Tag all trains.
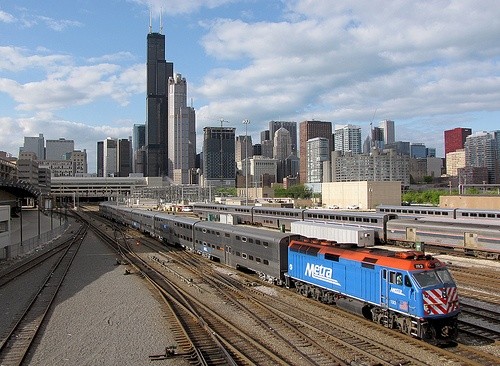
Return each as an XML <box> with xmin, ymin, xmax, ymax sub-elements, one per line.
<box><xmin>98</xmin><ymin>201</ymin><xmax>460</xmax><ymax>345</ymax></box>
<box><xmin>290</xmin><ymin>219</ymin><xmax>374</xmax><ymax>252</ymax></box>
<box><xmin>376</xmin><ymin>204</ymin><xmax>499</xmax><ymax>225</ymax></box>
<box><xmin>192</xmin><ymin>203</ymin><xmax>500</xmax><ymax>276</ymax></box>
